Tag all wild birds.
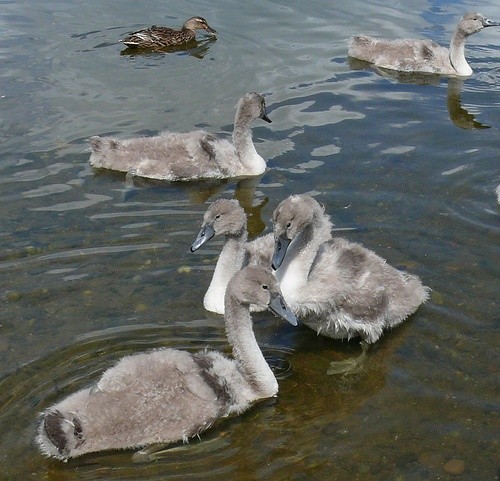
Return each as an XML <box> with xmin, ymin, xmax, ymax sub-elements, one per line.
<box><xmin>268</xmin><ymin>193</ymin><xmax>433</xmax><ymax>374</ymax></box>
<box><xmin>119</xmin><ymin>16</ymin><xmax>217</xmax><ymax>51</ymax></box>
<box><xmin>190</xmin><ymin>198</ymin><xmax>278</xmax><ymax>314</ymax></box>
<box><xmin>349</xmin><ymin>58</ymin><xmax>493</xmax><ymax>130</ymax></box>
<box><xmin>33</xmin><ymin>266</ymin><xmax>298</xmax><ymax>463</ymax></box>
<box><xmin>347</xmin><ymin>11</ymin><xmax>499</xmax><ymax>77</ymax></box>
<box><xmin>87</xmin><ymin>92</ymin><xmax>273</xmax><ymax>182</ymax></box>
